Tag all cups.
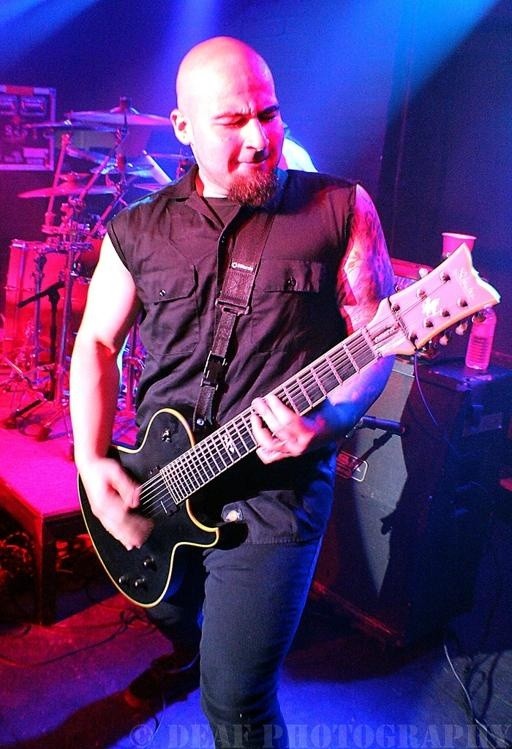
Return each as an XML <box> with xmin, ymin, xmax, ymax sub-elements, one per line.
<box><xmin>441</xmin><ymin>233</ymin><xmax>476</xmax><ymax>258</ymax></box>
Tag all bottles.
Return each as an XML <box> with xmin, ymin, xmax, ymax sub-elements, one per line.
<box><xmin>466</xmin><ymin>304</ymin><xmax>498</xmax><ymax>371</ymax></box>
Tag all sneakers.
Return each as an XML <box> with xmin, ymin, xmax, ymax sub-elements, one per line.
<box><xmin>123</xmin><ymin>649</ymin><xmax>200</xmax><ymax>709</ymax></box>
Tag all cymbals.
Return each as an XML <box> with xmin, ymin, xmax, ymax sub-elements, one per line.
<box><xmin>147</xmin><ymin>152</ymin><xmax>194</xmax><ymax>161</ymax></box>
<box><xmin>17</xmin><ymin>182</ymin><xmax>119</xmax><ymax>199</ymax></box>
<box><xmin>90</xmin><ymin>163</ymin><xmax>155</xmax><ymax>175</ymax></box>
<box><xmin>26</xmin><ymin>107</ymin><xmax>172</xmax><ymax>129</ymax></box>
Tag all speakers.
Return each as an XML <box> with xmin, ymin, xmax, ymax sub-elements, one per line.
<box><xmin>316</xmin><ymin>358</ymin><xmax>512</xmax><ymax>645</ymax></box>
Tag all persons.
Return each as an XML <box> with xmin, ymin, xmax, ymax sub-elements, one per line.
<box><xmin>69</xmin><ymin>35</ymin><xmax>395</xmax><ymax>749</ymax></box>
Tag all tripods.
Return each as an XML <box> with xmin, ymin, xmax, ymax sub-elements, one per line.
<box><xmin>0</xmin><ymin>156</ymin><xmax>116</xmax><ymax>462</ymax></box>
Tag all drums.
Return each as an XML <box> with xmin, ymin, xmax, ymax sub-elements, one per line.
<box><xmin>4</xmin><ymin>238</ymin><xmax>79</xmax><ymax>312</ymax></box>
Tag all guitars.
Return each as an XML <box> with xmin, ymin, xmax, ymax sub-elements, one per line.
<box><xmin>77</xmin><ymin>244</ymin><xmax>501</xmax><ymax>609</ymax></box>
